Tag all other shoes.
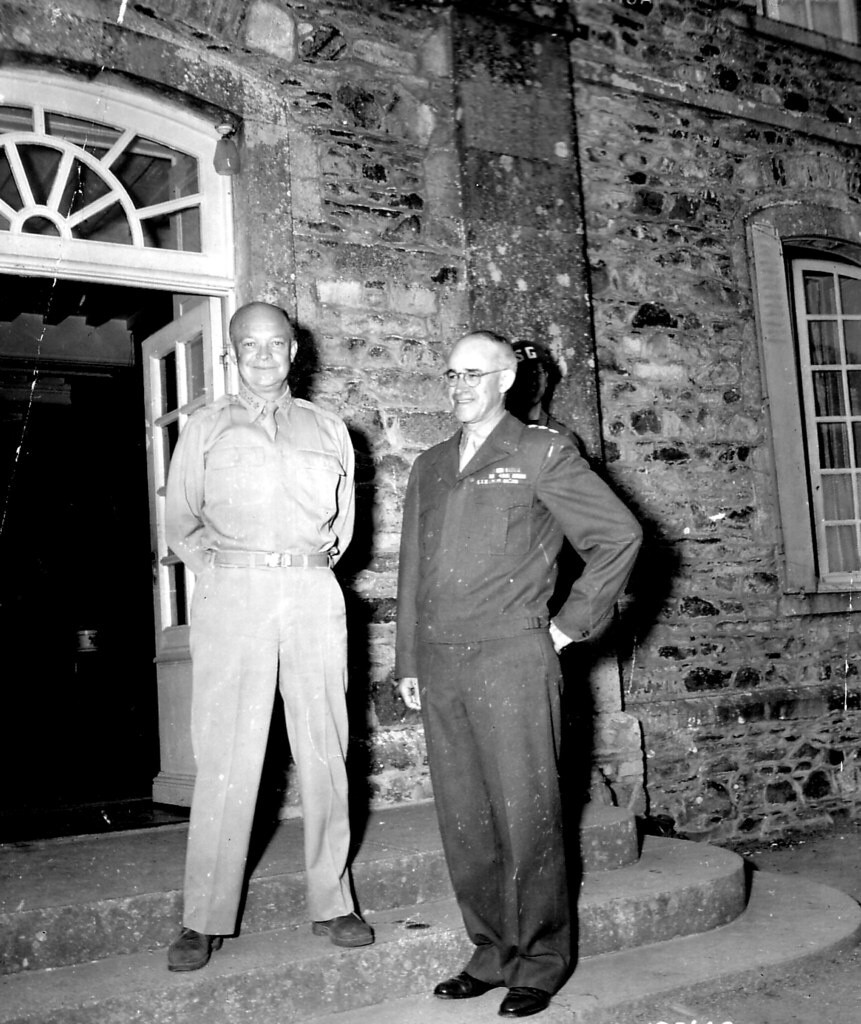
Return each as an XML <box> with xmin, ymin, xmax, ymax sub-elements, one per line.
<box><xmin>312</xmin><ymin>914</ymin><xmax>374</xmax><ymax>946</ymax></box>
<box><xmin>166</xmin><ymin>928</ymin><xmax>219</xmax><ymax>972</ymax></box>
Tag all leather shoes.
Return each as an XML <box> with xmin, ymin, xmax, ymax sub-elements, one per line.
<box><xmin>498</xmin><ymin>987</ymin><xmax>548</xmax><ymax>1017</ymax></box>
<box><xmin>432</xmin><ymin>971</ymin><xmax>493</xmax><ymax>999</ymax></box>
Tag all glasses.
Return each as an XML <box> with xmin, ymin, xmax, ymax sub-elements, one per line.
<box><xmin>441</xmin><ymin>369</ymin><xmax>505</xmax><ymax>387</ymax></box>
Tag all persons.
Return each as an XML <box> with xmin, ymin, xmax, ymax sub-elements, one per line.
<box><xmin>395</xmin><ymin>332</ymin><xmax>645</xmax><ymax>1014</ymax></box>
<box><xmin>165</xmin><ymin>302</ymin><xmax>374</xmax><ymax>973</ymax></box>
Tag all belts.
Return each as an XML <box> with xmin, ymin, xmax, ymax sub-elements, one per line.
<box><xmin>213</xmin><ymin>551</ymin><xmax>327</xmax><ymax>567</ymax></box>
